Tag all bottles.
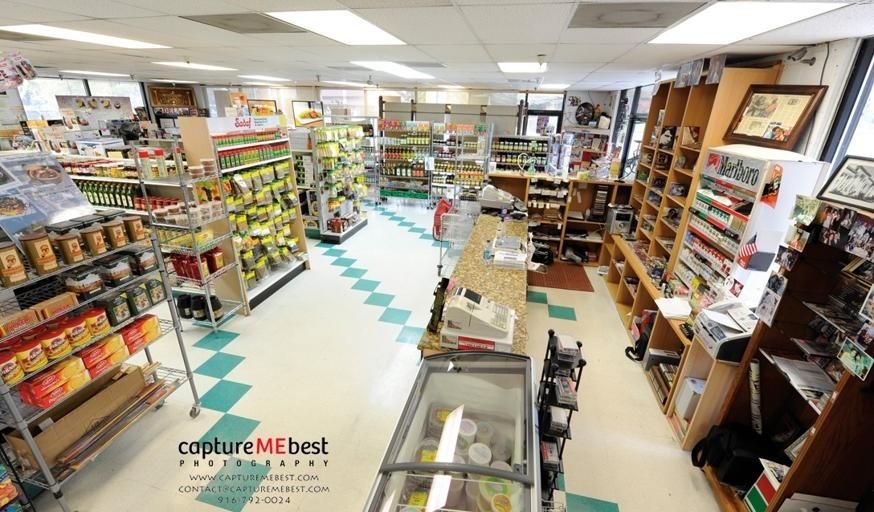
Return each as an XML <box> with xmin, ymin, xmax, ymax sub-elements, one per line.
<box><xmin>484</xmin><ymin>239</ymin><xmax>492</xmax><ymax>265</ymax></box>
<box><xmin>73</xmin><ymin>181</ymin><xmax>142</xmax><ymax>211</ymax></box>
<box><xmin>211</xmin><ymin>128</ymin><xmax>291</xmax><ymax>170</ymax></box>
<box><xmin>138</xmin><ymin>148</ymin><xmax>185</xmax><ymax>180</ymax></box>
<box><xmin>383</xmin><ymin>129</ymin><xmax>547</xmax><ymax>186</ymax></box>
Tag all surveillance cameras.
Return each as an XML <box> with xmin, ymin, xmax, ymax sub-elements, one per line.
<box><xmin>787</xmin><ymin>47</ymin><xmax>807</xmax><ymax>61</ymax></box>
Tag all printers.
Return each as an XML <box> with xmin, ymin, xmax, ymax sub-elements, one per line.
<box><xmin>691</xmin><ymin>298</ymin><xmax>760</xmax><ymax>362</ymax></box>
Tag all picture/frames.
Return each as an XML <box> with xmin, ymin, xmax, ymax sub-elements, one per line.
<box><xmin>148</xmin><ymin>86</ymin><xmax>199</xmax><ymax>108</ymax></box>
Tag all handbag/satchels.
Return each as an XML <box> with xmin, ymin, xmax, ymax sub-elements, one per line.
<box><xmin>636</xmin><ymin>322</ymin><xmax>654</xmax><ymax>359</ymax></box>
<box><xmin>565</xmin><ymin>244</ymin><xmax>589</xmax><ymax>264</ymax></box>
<box><xmin>532</xmin><ymin>247</ymin><xmax>553</xmax><ymax>265</ymax></box>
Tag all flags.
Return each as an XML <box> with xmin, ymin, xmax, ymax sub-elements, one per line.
<box><xmin>737</xmin><ymin>234</ymin><xmax>758</xmax><ymax>258</ymax></box>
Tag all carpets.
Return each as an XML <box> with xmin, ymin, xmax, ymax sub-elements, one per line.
<box><xmin>526</xmin><ymin>260</ymin><xmax>594</xmax><ymax>293</ymax></box>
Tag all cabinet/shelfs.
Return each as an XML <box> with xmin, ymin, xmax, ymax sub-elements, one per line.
<box><xmin>537</xmin><ymin>328</ymin><xmax>586</xmax><ymax>507</ymax></box>
<box><xmin>373</xmin><ymin>120</ymin><xmax>633</xmax><ymax>264</ymax></box>
<box><xmin>598</xmin><ymin>63</ymin><xmax>874</xmax><ymax>511</ymax></box>
<box><xmin>0</xmin><ymin>114</ymin><xmax>366</xmax><ymax>511</ymax></box>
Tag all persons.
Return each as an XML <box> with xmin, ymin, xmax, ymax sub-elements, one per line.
<box><xmin>764</xmin><ymin>126</ymin><xmax>789</xmax><ymax>142</ymax></box>
<box><xmin>659</xmin><ymin>126</ymin><xmax>676</xmax><ymax>150</ymax></box>
<box><xmin>753</xmin><ymin>210</ymin><xmax>874</xmax><ymax>378</ymax></box>
<box><xmin>665</xmin><ymin>209</ymin><xmax>677</xmax><ymax>219</ymax></box>
<box><xmin>746</xmin><ymin>96</ymin><xmax>778</xmax><ymax>118</ymax></box>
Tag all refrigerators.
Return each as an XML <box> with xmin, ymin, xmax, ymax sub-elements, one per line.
<box><xmin>366</xmin><ymin>349</ymin><xmax>538</xmax><ymax>512</ymax></box>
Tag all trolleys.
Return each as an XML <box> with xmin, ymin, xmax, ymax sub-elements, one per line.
<box><xmin>437</xmin><ymin>176</ymin><xmax>486</xmax><ymax>276</ymax></box>
<box><xmin>374</xmin><ymin>162</ymin><xmax>430</xmax><ymax>209</ymax></box>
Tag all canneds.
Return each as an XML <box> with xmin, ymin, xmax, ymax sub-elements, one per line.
<box><xmin>0</xmin><ymin>241</ymin><xmax>29</xmax><ymax>289</ymax></box>
<box><xmin>101</xmin><ymin>220</ymin><xmax>127</xmax><ymax>249</ymax></box>
<box><xmin>122</xmin><ymin>216</ymin><xmax>145</xmax><ymax>242</ymax></box>
<box><xmin>17</xmin><ymin>233</ymin><xmax>59</xmax><ymax>275</ymax></box>
<box><xmin>54</xmin><ymin>234</ymin><xmax>85</xmax><ymax>265</ymax></box>
<box><xmin>491</xmin><ymin>141</ymin><xmax>547</xmax><ymax>164</ymax></box>
<box><xmin>1</xmin><ymin>307</ymin><xmax>111</xmax><ymax>383</ymax></box>
<box><xmin>133</xmin><ymin>196</ymin><xmax>180</xmax><ymax>212</ymax></box>
<box><xmin>79</xmin><ymin>226</ymin><xmax>108</xmax><ymax>257</ymax></box>
